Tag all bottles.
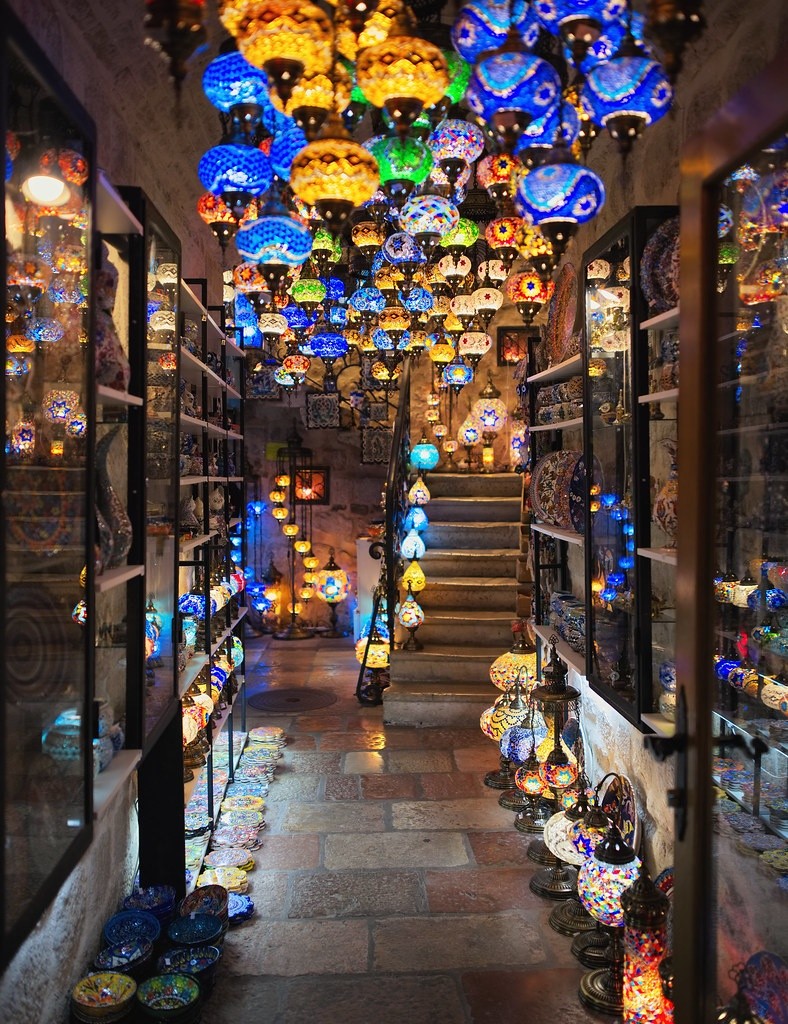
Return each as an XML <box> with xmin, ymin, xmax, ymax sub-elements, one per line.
<box><xmin>731</xmin><ymin>569</ymin><xmax>758</xmax><ymax>607</ymax></box>
<box><xmin>728</xmin><ymin>658</ymin><xmax>757</xmax><ymax>687</ymax></box>
<box><xmin>761</xmin><ymin>670</ymin><xmax>788</xmax><ymax>710</ymax></box>
<box><xmin>712</xmin><ymin>567</ymin><xmax>724</xmax><ymax>585</ymax></box>
<box><xmin>743</xmin><ymin>656</ymin><xmax>777</xmax><ymax>697</ymax></box>
<box><xmin>763</xmin><ymin>627</ymin><xmax>781</xmax><ymax>643</ymax></box>
<box><xmin>715</xmin><ymin>569</ymin><xmax>739</xmax><ymax>603</ymax></box>
<box><xmin>751</xmin><ymin>618</ymin><xmax>771</xmax><ymax>643</ymax></box>
<box><xmin>715</xmin><ymin>649</ymin><xmax>741</xmax><ymax>681</ymax></box>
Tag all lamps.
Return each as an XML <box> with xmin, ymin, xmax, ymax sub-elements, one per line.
<box><xmin>0</xmin><ymin>0</ymin><xmax>788</xmax><ymax>1024</ymax></box>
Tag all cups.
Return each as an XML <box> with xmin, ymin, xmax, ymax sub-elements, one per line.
<box><xmin>43</xmin><ymin>700</ymin><xmax>125</xmax><ymax>780</ymax></box>
<box><xmin>537</xmin><ymin>375</ymin><xmax>586</xmax><ymax>423</ymax></box>
<box><xmin>659</xmin><ymin>331</ymin><xmax>681</xmax><ymax>389</ymax></box>
<box><xmin>658</xmin><ymin>661</ymin><xmax>677</xmax><ymax>722</ymax></box>
<box><xmin>549</xmin><ymin>590</ymin><xmax>616</xmax><ymax>676</ymax></box>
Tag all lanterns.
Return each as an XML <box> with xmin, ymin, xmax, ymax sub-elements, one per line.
<box><xmin>143</xmin><ymin>0</ymin><xmax>788</xmax><ymax>1024</ymax></box>
<box><xmin>5</xmin><ymin>129</ymin><xmax>92</xmax><ymax>455</ymax></box>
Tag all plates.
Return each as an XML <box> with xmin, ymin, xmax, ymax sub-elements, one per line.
<box><xmin>542</xmin><ymin>262</ymin><xmax>582</xmax><ymax>366</ymax></box>
<box><xmin>640</xmin><ymin>215</ymin><xmax>680</xmax><ymax>311</ymax></box>
<box><xmin>530</xmin><ymin>451</ymin><xmax>606</xmax><ymax>535</ymax></box>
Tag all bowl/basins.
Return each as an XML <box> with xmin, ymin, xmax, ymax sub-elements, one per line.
<box><xmin>71</xmin><ymin>884</ymin><xmax>229</xmax><ymax>1024</ymax></box>
